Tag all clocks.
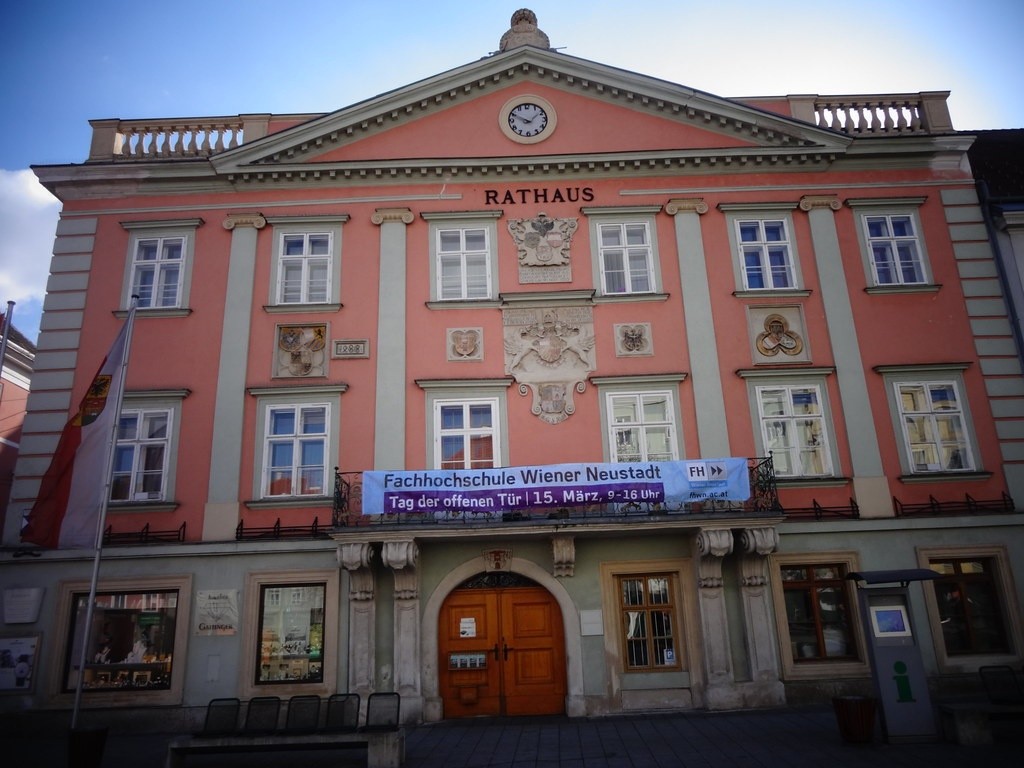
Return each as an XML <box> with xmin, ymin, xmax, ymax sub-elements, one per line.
<box><xmin>498</xmin><ymin>94</ymin><xmax>558</xmax><ymax>144</ymax></box>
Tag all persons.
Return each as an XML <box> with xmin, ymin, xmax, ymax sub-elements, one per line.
<box><xmin>0</xmin><ymin>649</ymin><xmax>16</xmax><ymax>669</ymax></box>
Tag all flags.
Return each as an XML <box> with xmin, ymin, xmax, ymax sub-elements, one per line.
<box><xmin>20</xmin><ymin>308</ymin><xmax>134</xmax><ymax>548</ymax></box>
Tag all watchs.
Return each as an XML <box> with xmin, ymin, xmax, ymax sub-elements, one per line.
<box><xmin>15</xmin><ymin>654</ymin><xmax>29</xmax><ymax>687</ymax></box>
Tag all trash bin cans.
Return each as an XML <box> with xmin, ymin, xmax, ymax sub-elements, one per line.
<box><xmin>833</xmin><ymin>695</ymin><xmax>878</xmax><ymax>745</ymax></box>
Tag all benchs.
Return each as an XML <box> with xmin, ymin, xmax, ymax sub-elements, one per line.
<box><xmin>936</xmin><ymin>664</ymin><xmax>1024</xmax><ymax>747</ymax></box>
<box><xmin>167</xmin><ymin>692</ymin><xmax>406</xmax><ymax>768</ymax></box>
